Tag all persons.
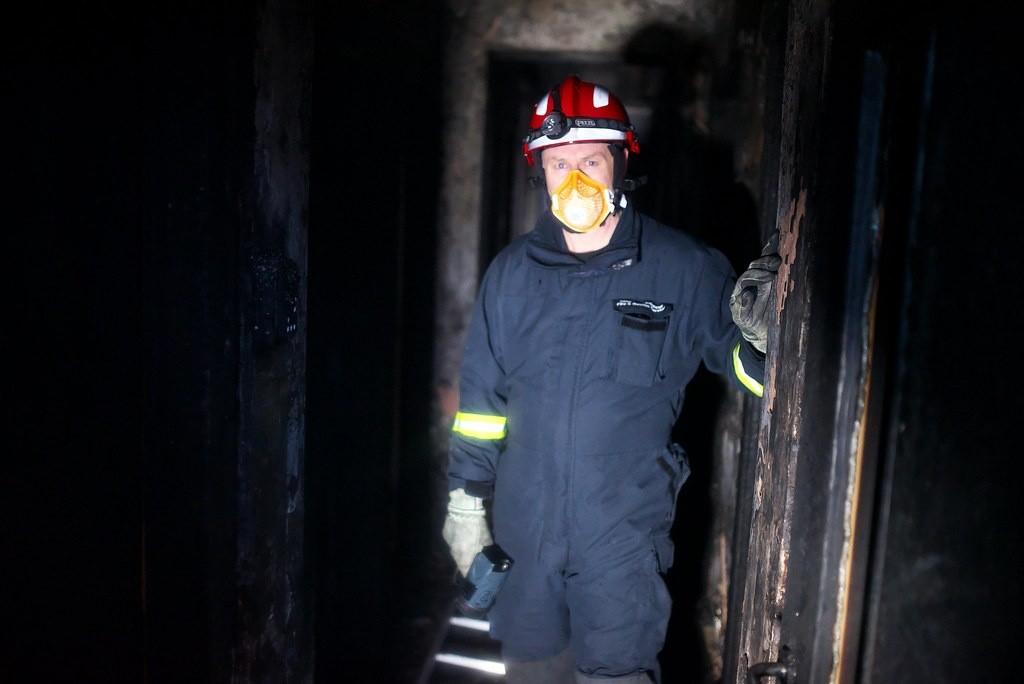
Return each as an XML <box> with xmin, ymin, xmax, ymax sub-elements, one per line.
<box><xmin>441</xmin><ymin>74</ymin><xmax>782</xmax><ymax>684</ymax></box>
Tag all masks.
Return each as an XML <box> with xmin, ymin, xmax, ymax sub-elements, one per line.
<box><xmin>551</xmin><ymin>169</ymin><xmax>616</xmax><ymax>234</ymax></box>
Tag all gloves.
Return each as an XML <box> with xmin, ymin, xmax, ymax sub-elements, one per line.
<box><xmin>730</xmin><ymin>230</ymin><xmax>783</xmax><ymax>351</ymax></box>
<box><xmin>441</xmin><ymin>486</ymin><xmax>494</xmax><ymax>583</ymax></box>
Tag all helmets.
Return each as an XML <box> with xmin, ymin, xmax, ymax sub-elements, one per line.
<box><xmin>523</xmin><ymin>75</ymin><xmax>639</xmax><ymax>165</ymax></box>
